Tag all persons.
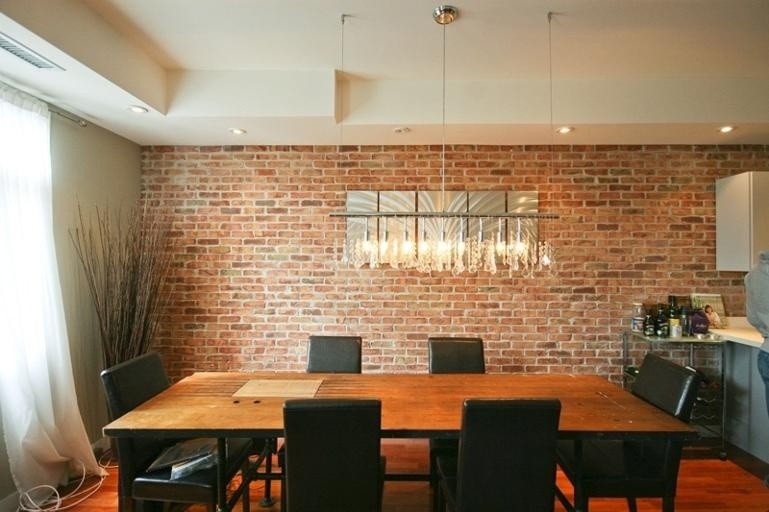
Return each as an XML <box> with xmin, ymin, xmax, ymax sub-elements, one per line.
<box><xmin>705</xmin><ymin>304</ymin><xmax>721</xmax><ymax>328</ymax></box>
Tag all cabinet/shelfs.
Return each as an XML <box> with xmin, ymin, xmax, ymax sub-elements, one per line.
<box><xmin>625</xmin><ymin>332</ymin><xmax>728</xmax><ymax>458</ymax></box>
<box><xmin>725</xmin><ymin>346</ymin><xmax>768</xmax><ymax>479</ymax></box>
<box><xmin>714</xmin><ymin>170</ymin><xmax>769</xmax><ymax>274</ymax></box>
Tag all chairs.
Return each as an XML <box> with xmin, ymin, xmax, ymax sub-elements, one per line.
<box><xmin>435</xmin><ymin>400</ymin><xmax>562</xmax><ymax>511</ymax></box>
<box><xmin>282</xmin><ymin>398</ymin><xmax>385</xmax><ymax>510</ymax></box>
<box><xmin>103</xmin><ymin>351</ymin><xmax>253</xmax><ymax>512</ymax></box>
<box><xmin>428</xmin><ymin>338</ymin><xmax>485</xmax><ymax>487</ymax></box>
<box><xmin>556</xmin><ymin>353</ymin><xmax>699</xmax><ymax>511</ymax></box>
<box><xmin>306</xmin><ymin>336</ymin><xmax>362</xmax><ymax>373</ymax></box>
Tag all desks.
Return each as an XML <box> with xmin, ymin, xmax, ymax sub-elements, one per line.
<box><xmin>104</xmin><ymin>372</ymin><xmax>698</xmax><ymax>511</ymax></box>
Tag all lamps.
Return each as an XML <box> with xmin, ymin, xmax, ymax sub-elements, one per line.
<box><xmin>331</xmin><ymin>5</ymin><xmax>557</xmax><ymax>279</ymax></box>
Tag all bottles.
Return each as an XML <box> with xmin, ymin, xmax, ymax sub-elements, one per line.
<box><xmin>631</xmin><ymin>295</ymin><xmax>692</xmax><ymax>338</ymax></box>
